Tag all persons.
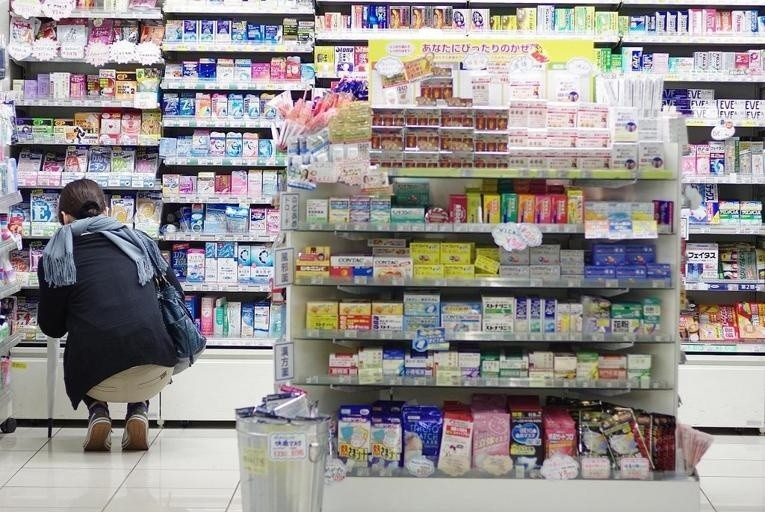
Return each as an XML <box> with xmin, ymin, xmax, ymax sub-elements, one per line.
<box><xmin>37</xmin><ymin>178</ymin><xmax>186</xmax><ymax>452</ymax></box>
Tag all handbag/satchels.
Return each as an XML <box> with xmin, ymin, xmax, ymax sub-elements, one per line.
<box><xmin>133</xmin><ymin>230</ymin><xmax>206</xmax><ymax>374</ymax></box>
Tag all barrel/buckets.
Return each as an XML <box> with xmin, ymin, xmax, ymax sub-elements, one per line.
<box><xmin>236</xmin><ymin>416</ymin><xmax>332</xmax><ymax>512</ymax></box>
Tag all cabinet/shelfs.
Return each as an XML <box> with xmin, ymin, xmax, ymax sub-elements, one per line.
<box><xmin>0</xmin><ymin>2</ymin><xmax>764</xmax><ymax>476</ymax></box>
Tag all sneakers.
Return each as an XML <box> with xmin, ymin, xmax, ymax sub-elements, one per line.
<box><xmin>122</xmin><ymin>410</ymin><xmax>149</xmax><ymax>450</ymax></box>
<box><xmin>83</xmin><ymin>407</ymin><xmax>112</xmax><ymax>451</ymax></box>
